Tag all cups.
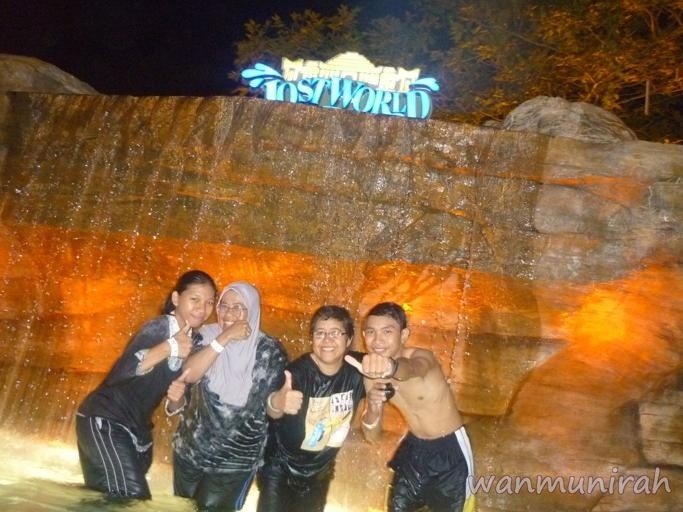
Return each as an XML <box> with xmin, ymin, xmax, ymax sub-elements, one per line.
<box><xmin>216</xmin><ymin>305</ymin><xmax>247</xmax><ymax>314</ymax></box>
<box><xmin>313</xmin><ymin>330</ymin><xmax>349</xmax><ymax>339</ymax></box>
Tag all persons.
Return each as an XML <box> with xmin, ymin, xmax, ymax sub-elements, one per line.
<box><xmin>254</xmin><ymin>306</ymin><xmax>379</xmax><ymax>510</ymax></box>
<box><xmin>358</xmin><ymin>301</ymin><xmax>476</xmax><ymax>510</ymax></box>
<box><xmin>74</xmin><ymin>270</ymin><xmax>216</xmax><ymax>504</ymax></box>
<box><xmin>166</xmin><ymin>281</ymin><xmax>289</xmax><ymax>511</ymax></box>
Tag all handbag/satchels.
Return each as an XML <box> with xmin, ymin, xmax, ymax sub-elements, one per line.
<box><xmin>267</xmin><ymin>390</ymin><xmax>277</xmax><ymax>413</ymax></box>
<box><xmin>383</xmin><ymin>357</ymin><xmax>399</xmax><ymax>378</ymax></box>
<box><xmin>211</xmin><ymin>339</ymin><xmax>221</xmax><ymax>353</ymax></box>
<box><xmin>361</xmin><ymin>409</ymin><xmax>380</xmax><ymax>429</ymax></box>
<box><xmin>168</xmin><ymin>336</ymin><xmax>177</xmax><ymax>357</ymax></box>
